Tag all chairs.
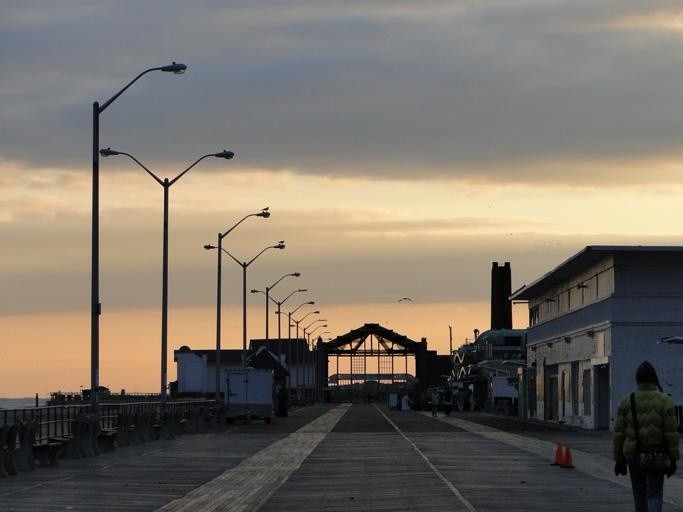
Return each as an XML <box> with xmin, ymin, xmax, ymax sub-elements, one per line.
<box><xmin>0</xmin><ymin>404</ymin><xmax>225</xmax><ymax>477</ymax></box>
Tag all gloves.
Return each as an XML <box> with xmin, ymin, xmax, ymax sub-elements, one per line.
<box><xmin>666</xmin><ymin>461</ymin><xmax>679</xmax><ymax>480</ymax></box>
<box><xmin>614</xmin><ymin>461</ymin><xmax>629</xmax><ymax>477</ymax></box>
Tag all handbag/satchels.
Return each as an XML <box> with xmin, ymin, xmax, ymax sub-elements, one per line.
<box><xmin>633</xmin><ymin>446</ymin><xmax>674</xmax><ymax>474</ymax></box>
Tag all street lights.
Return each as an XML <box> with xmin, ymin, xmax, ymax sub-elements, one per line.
<box><xmin>203</xmin><ymin>242</ymin><xmax>284</xmax><ymax>361</ymax></box>
<box><xmin>97</xmin><ymin>147</ymin><xmax>235</xmax><ymax>420</ymax></box>
<box><xmin>212</xmin><ymin>208</ymin><xmax>271</xmax><ymax>412</ymax></box>
<box><xmin>87</xmin><ymin>62</ymin><xmax>186</xmax><ymax>416</ymax></box>
<box><xmin>265</xmin><ymin>272</ymin><xmax>301</xmax><ymax>341</ymax></box>
<box><xmin>250</xmin><ymin>287</ymin><xmax>332</xmax><ymax>352</ymax></box>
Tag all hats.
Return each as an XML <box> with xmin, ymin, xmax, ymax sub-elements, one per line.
<box><xmin>635</xmin><ymin>360</ymin><xmax>658</xmax><ymax>383</ymax></box>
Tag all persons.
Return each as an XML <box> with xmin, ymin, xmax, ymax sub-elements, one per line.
<box><xmin>276</xmin><ymin>389</ymin><xmax>290</xmax><ymax>416</ymax></box>
<box><xmin>431</xmin><ymin>387</ymin><xmax>466</xmax><ymax>418</ymax></box>
<box><xmin>612</xmin><ymin>359</ymin><xmax>679</xmax><ymax>512</ymax></box>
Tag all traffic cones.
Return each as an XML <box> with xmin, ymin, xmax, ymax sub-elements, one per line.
<box><xmin>551</xmin><ymin>443</ymin><xmax>566</xmax><ymax>465</ymax></box>
<box><xmin>560</xmin><ymin>446</ymin><xmax>575</xmax><ymax>468</ymax></box>
